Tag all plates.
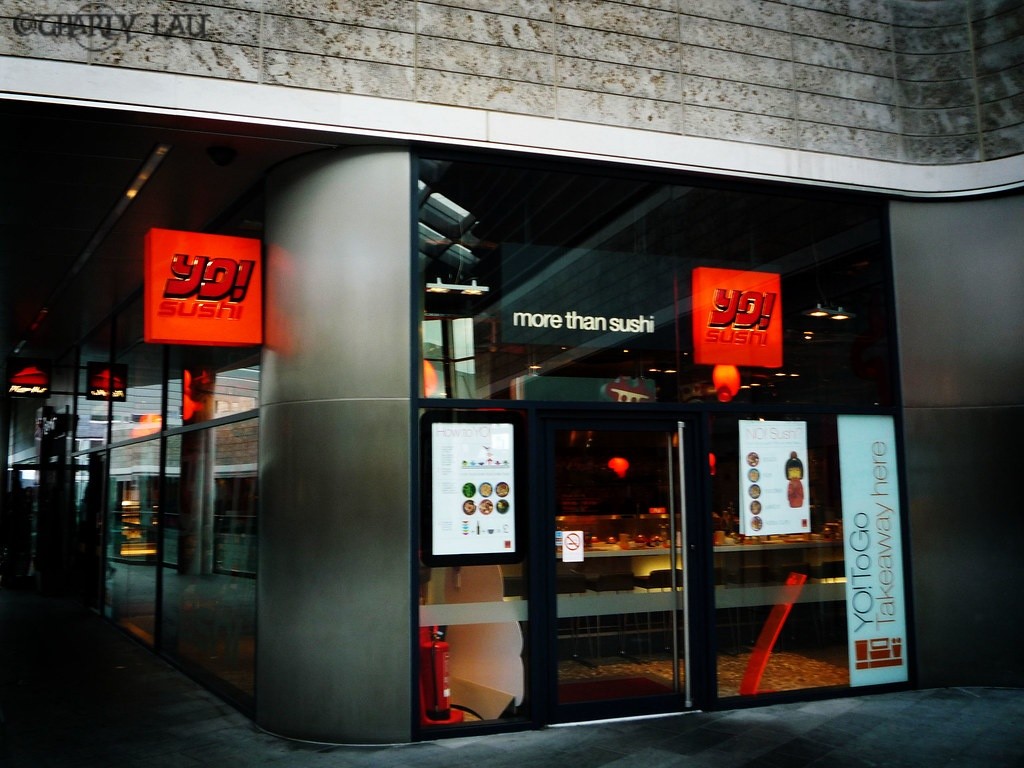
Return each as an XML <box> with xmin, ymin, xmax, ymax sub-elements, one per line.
<box><xmin>761</xmin><ymin>540</ymin><xmax>783</xmax><ymax>543</ymax></box>
<box><xmin>721</xmin><ymin>544</ymin><xmax>736</xmax><ymax>546</ymax></box>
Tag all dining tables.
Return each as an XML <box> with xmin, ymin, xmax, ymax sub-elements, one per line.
<box><xmin>555</xmin><ymin>519</ymin><xmax>844</xmax><ymax>560</ymax></box>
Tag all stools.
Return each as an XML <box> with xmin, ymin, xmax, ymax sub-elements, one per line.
<box><xmin>518</xmin><ymin>561</ymin><xmax>845</xmax><ymax>667</ymax></box>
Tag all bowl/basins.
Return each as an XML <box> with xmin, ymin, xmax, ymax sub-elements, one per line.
<box><xmin>724</xmin><ymin>538</ymin><xmax>733</xmax><ymax>544</ymax></box>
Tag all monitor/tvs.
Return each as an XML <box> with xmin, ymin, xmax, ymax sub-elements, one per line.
<box><xmin>426</xmin><ymin>241</ymin><xmax>507</xmax><ymax>317</ymax></box>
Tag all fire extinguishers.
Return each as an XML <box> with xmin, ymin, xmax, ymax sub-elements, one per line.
<box><xmin>421</xmin><ymin>630</ymin><xmax>450</xmax><ymax>711</ymax></box>
